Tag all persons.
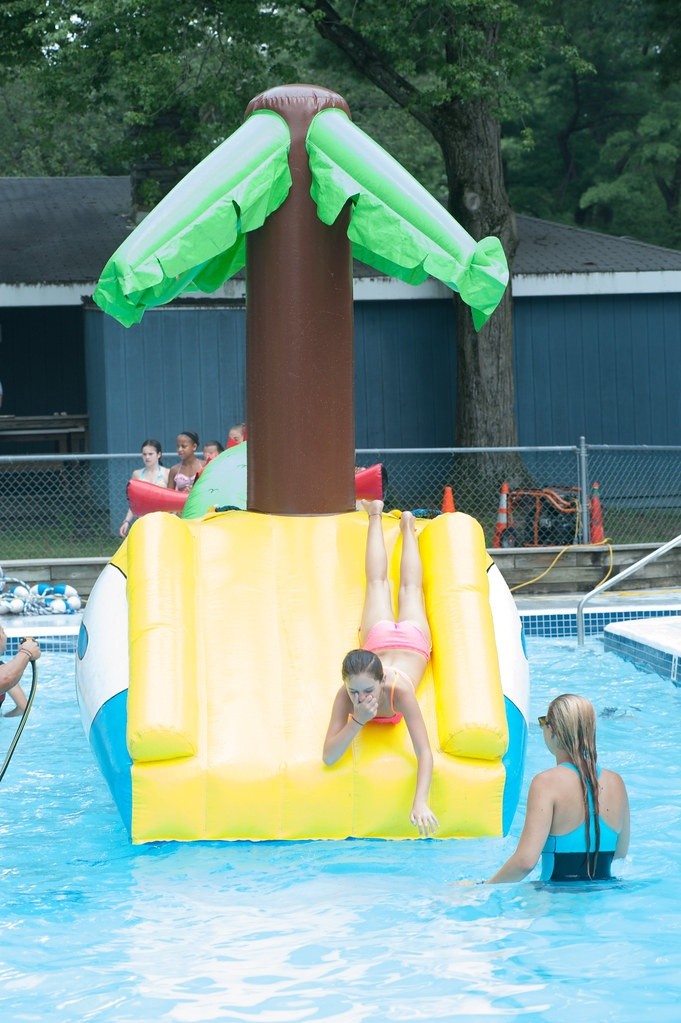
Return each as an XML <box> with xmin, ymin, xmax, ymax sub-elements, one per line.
<box><xmin>322</xmin><ymin>496</ymin><xmax>439</xmax><ymax>838</ymax></box>
<box><xmin>119</xmin><ymin>440</ymin><xmax>179</xmax><ymax>538</ymax></box>
<box><xmin>0</xmin><ymin>627</ymin><xmax>42</xmax><ymax>719</ymax></box>
<box><xmin>203</xmin><ymin>422</ymin><xmax>247</xmax><ymax>462</ymax></box>
<box><xmin>167</xmin><ymin>431</ymin><xmax>206</xmax><ymax>494</ymax></box>
<box><xmin>475</xmin><ymin>694</ymin><xmax>631</xmax><ymax>885</ymax></box>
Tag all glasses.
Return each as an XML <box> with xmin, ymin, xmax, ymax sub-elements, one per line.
<box><xmin>538</xmin><ymin>716</ymin><xmax>556</xmax><ymax>734</ymax></box>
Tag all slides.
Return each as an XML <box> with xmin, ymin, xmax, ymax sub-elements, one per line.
<box><xmin>74</xmin><ymin>509</ymin><xmax>533</xmax><ymax>845</ymax></box>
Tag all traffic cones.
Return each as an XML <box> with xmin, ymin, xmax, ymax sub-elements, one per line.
<box><xmin>591</xmin><ymin>481</ymin><xmax>606</xmax><ymax>545</ymax></box>
<box><xmin>493</xmin><ymin>482</ymin><xmax>510</xmax><ymax>550</ymax></box>
<box><xmin>442</xmin><ymin>485</ymin><xmax>455</xmax><ymax>514</ymax></box>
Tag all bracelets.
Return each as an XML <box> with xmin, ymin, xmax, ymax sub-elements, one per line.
<box><xmin>20</xmin><ymin>648</ymin><xmax>33</xmax><ymax>657</ymax></box>
<box><xmin>122</xmin><ymin>520</ymin><xmax>130</xmax><ymax>525</ymax></box>
<box><xmin>352</xmin><ymin>716</ymin><xmax>364</xmax><ymax>726</ymax></box>
<box><xmin>480</xmin><ymin>878</ymin><xmax>485</xmax><ymax>885</ymax></box>
